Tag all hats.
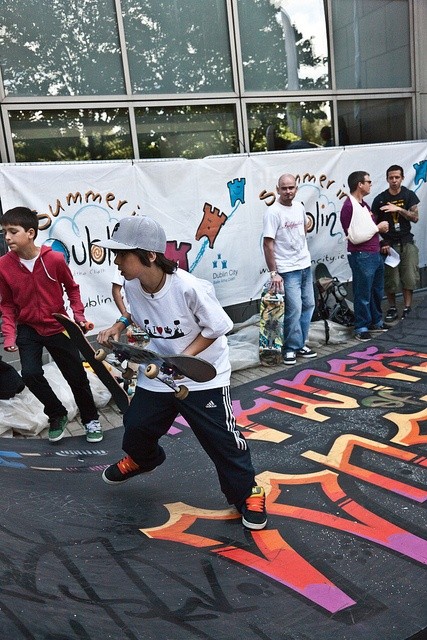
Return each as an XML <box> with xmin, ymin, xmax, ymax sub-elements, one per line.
<box><xmin>91</xmin><ymin>215</ymin><xmax>167</xmax><ymax>255</ymax></box>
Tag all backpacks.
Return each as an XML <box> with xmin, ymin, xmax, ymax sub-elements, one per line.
<box><xmin>314</xmin><ymin>276</ymin><xmax>355</xmax><ymax>345</ymax></box>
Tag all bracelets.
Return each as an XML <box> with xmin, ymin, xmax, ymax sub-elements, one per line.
<box><xmin>115</xmin><ymin>316</ymin><xmax>130</xmax><ymax>329</ymax></box>
<box><xmin>270</xmin><ymin>271</ymin><xmax>278</xmax><ymax>276</ymax></box>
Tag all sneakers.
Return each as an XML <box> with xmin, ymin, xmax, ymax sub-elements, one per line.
<box><xmin>401</xmin><ymin>306</ymin><xmax>412</xmax><ymax>320</ymax></box>
<box><xmin>355</xmin><ymin>332</ymin><xmax>372</xmax><ymax>342</ymax></box>
<box><xmin>296</xmin><ymin>345</ymin><xmax>318</xmax><ymax>358</ymax></box>
<box><xmin>84</xmin><ymin>419</ymin><xmax>104</xmax><ymax>443</ymax></box>
<box><xmin>47</xmin><ymin>414</ymin><xmax>69</xmax><ymax>443</ymax></box>
<box><xmin>101</xmin><ymin>454</ymin><xmax>159</xmax><ymax>486</ymax></box>
<box><xmin>282</xmin><ymin>351</ymin><xmax>297</xmax><ymax>365</ymax></box>
<box><xmin>385</xmin><ymin>306</ymin><xmax>398</xmax><ymax>323</ymax></box>
<box><xmin>368</xmin><ymin>322</ymin><xmax>393</xmax><ymax>333</ymax></box>
<box><xmin>241</xmin><ymin>486</ymin><xmax>268</xmax><ymax>531</ymax></box>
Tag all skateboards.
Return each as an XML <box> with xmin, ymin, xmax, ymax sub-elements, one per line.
<box><xmin>258</xmin><ymin>279</ymin><xmax>285</xmax><ymax>367</ymax></box>
<box><xmin>51</xmin><ymin>313</ymin><xmax>130</xmax><ymax>413</ymax></box>
<box><xmin>314</xmin><ymin>263</ymin><xmax>354</xmax><ymax>328</ymax></box>
<box><xmin>94</xmin><ymin>337</ymin><xmax>216</xmax><ymax>401</ymax></box>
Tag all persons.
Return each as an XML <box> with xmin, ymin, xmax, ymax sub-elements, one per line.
<box><xmin>96</xmin><ymin>214</ymin><xmax>268</xmax><ymax>530</ymax></box>
<box><xmin>371</xmin><ymin>164</ymin><xmax>419</xmax><ymax>323</ymax></box>
<box><xmin>110</xmin><ymin>267</ymin><xmax>149</xmax><ymax>393</ymax></box>
<box><xmin>263</xmin><ymin>173</ymin><xmax>318</xmax><ymax>364</ymax></box>
<box><xmin>0</xmin><ymin>206</ymin><xmax>104</xmax><ymax>443</ymax></box>
<box><xmin>339</xmin><ymin>171</ymin><xmax>393</xmax><ymax>342</ymax></box>
